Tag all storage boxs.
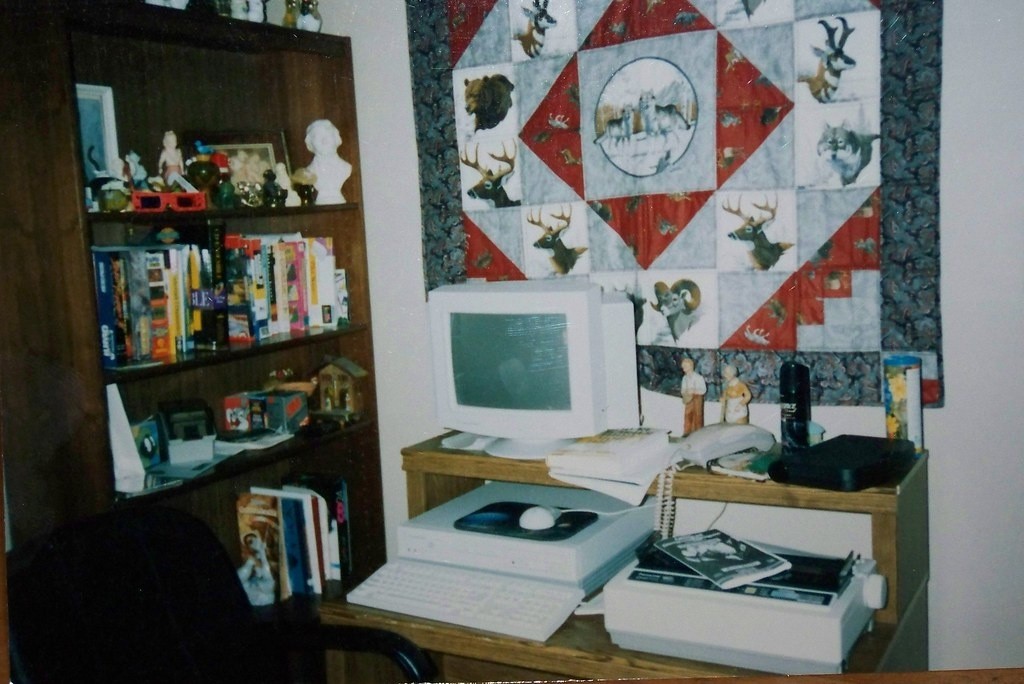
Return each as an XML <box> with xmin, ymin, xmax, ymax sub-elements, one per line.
<box><xmin>156</xmin><ymin>408</ymin><xmax>217</xmax><ymax>464</ymax></box>
<box><xmin>250</xmin><ymin>389</ymin><xmax>310</xmax><ymax>434</ymax></box>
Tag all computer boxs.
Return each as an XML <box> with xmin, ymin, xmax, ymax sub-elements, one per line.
<box><xmin>396</xmin><ymin>481</ymin><xmax>657</xmax><ymax>596</ymax></box>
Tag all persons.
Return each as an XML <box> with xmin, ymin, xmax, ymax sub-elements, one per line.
<box><xmin>681</xmin><ymin>357</ymin><xmax>751</xmax><ymax>438</ymax></box>
<box><xmin>159</xmin><ymin>118</ymin><xmax>353</xmax><ymax>205</ymax></box>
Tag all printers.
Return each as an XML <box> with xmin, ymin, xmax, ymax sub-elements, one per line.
<box><xmin>603</xmin><ymin>540</ymin><xmax>888</xmax><ymax>675</ymax></box>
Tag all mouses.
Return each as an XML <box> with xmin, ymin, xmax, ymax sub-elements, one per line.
<box><xmin>520</xmin><ymin>504</ymin><xmax>562</xmax><ymax>530</ymax></box>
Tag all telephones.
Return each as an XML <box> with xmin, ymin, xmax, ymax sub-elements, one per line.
<box><xmin>680</xmin><ymin>422</ymin><xmax>776</xmax><ymax>470</ymax></box>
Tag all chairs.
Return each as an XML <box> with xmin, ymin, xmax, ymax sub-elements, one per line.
<box><xmin>5</xmin><ymin>502</ymin><xmax>439</xmax><ymax>684</ymax></box>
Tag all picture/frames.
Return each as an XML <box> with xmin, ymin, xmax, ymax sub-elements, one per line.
<box><xmin>186</xmin><ymin>129</ymin><xmax>292</xmax><ymax>191</ymax></box>
<box><xmin>77</xmin><ymin>83</ymin><xmax>119</xmax><ymax>181</ymax></box>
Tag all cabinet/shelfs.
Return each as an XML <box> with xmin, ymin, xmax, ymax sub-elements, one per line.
<box><xmin>0</xmin><ymin>0</ymin><xmax>388</xmax><ymax>621</ymax></box>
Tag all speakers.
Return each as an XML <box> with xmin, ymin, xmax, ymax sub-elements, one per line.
<box><xmin>601</xmin><ymin>292</ymin><xmax>641</xmax><ymax>431</ymax></box>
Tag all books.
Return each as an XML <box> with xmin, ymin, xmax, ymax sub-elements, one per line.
<box><xmin>653</xmin><ymin>528</ymin><xmax>793</xmax><ymax>591</ymax></box>
<box><xmin>91</xmin><ymin>232</ymin><xmax>348</xmax><ymax>605</ymax></box>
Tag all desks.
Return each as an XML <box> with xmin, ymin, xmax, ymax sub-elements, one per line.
<box><xmin>314</xmin><ymin>430</ymin><xmax>932</xmax><ymax>681</ymax></box>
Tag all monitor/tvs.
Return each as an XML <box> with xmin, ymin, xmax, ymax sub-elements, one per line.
<box><xmin>428</xmin><ymin>279</ymin><xmax>608</xmax><ymax>460</ymax></box>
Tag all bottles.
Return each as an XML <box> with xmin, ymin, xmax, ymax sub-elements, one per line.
<box><xmin>780</xmin><ymin>360</ymin><xmax>811</xmax><ymax>459</ymax></box>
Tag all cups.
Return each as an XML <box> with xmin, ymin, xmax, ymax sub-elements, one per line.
<box><xmin>883</xmin><ymin>355</ymin><xmax>923</xmax><ymax>458</ymax></box>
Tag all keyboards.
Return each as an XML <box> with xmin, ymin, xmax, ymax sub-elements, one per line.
<box><xmin>346</xmin><ymin>557</ymin><xmax>585</xmax><ymax>641</ymax></box>
